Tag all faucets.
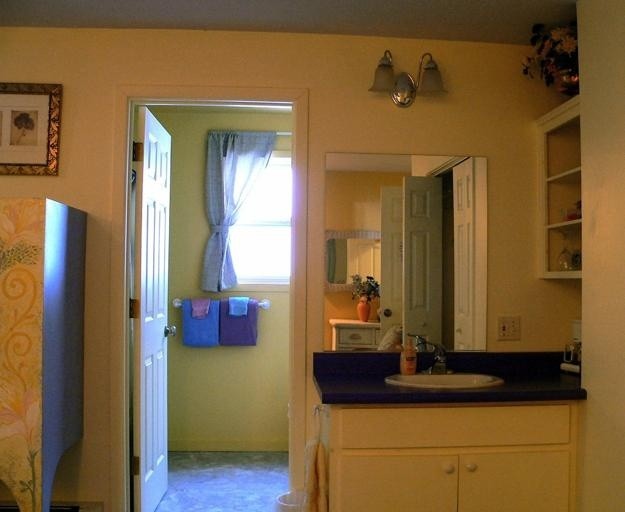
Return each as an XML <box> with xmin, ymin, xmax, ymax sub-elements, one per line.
<box><xmin>407</xmin><ymin>332</ymin><xmax>431</xmax><ymax>353</ymax></box>
<box><xmin>415</xmin><ymin>341</ymin><xmax>453</xmax><ymax>376</ymax></box>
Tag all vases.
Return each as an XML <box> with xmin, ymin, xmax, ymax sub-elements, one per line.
<box><xmin>357</xmin><ymin>297</ymin><xmax>372</xmax><ymax>323</ymax></box>
<box><xmin>552</xmin><ymin>69</ymin><xmax>581</xmax><ymax>97</ymax></box>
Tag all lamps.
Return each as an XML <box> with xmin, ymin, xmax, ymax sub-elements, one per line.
<box><xmin>366</xmin><ymin>48</ymin><xmax>450</xmax><ymax>109</ymax></box>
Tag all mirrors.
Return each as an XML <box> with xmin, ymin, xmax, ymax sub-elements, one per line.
<box><xmin>322</xmin><ymin>150</ymin><xmax>488</xmax><ymax>352</ymax></box>
<box><xmin>324</xmin><ymin>230</ymin><xmax>380</xmax><ymax>293</ymax></box>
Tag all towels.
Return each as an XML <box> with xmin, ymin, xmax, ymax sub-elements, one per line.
<box><xmin>183</xmin><ymin>296</ymin><xmax>261</xmax><ymax>350</ymax></box>
<box><xmin>300</xmin><ymin>439</ymin><xmax>331</xmax><ymax>512</ymax></box>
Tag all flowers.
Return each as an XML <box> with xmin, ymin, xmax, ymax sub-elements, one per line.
<box><xmin>520</xmin><ymin>13</ymin><xmax>581</xmax><ymax>97</ymax></box>
<box><xmin>350</xmin><ymin>274</ymin><xmax>384</xmax><ymax>305</ymax></box>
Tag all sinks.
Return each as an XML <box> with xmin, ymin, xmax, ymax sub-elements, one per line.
<box><xmin>384</xmin><ymin>372</ymin><xmax>504</xmax><ymax>390</ymax></box>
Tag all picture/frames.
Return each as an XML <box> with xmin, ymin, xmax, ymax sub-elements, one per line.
<box><xmin>0</xmin><ymin>81</ymin><xmax>63</xmax><ymax>177</ymax></box>
<box><xmin>535</xmin><ymin>96</ymin><xmax>583</xmax><ymax>281</ymax></box>
<box><xmin>331</xmin><ymin>403</ymin><xmax>586</xmax><ymax>512</ymax></box>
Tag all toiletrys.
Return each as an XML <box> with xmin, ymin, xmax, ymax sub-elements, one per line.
<box><xmin>399</xmin><ymin>337</ymin><xmax>418</xmax><ymax>375</ymax></box>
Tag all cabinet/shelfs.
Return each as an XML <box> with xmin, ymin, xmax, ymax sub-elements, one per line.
<box><xmin>329</xmin><ymin>318</ymin><xmax>384</xmax><ymax>353</ymax></box>
